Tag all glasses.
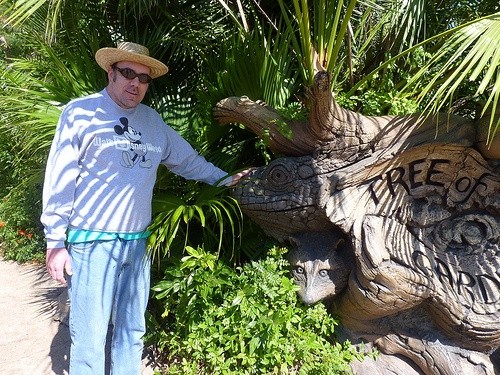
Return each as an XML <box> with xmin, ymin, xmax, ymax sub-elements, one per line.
<box><xmin>112</xmin><ymin>66</ymin><xmax>153</xmax><ymax>84</ymax></box>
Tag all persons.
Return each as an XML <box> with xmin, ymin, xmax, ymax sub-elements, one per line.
<box><xmin>40</xmin><ymin>42</ymin><xmax>258</xmax><ymax>375</ymax></box>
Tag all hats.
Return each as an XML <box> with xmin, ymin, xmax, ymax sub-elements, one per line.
<box><xmin>95</xmin><ymin>42</ymin><xmax>169</xmax><ymax>79</ymax></box>
